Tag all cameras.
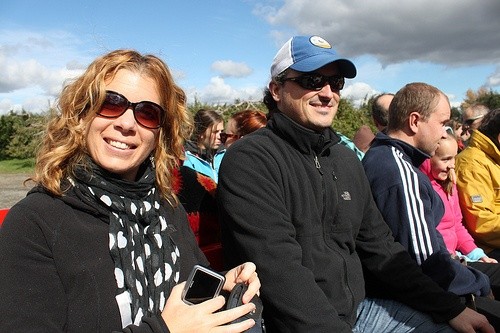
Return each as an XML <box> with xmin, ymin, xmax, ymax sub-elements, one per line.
<box><xmin>182</xmin><ymin>264</ymin><xmax>226</xmax><ymax>306</ymax></box>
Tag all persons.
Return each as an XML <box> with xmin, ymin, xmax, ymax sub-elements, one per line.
<box><xmin>0</xmin><ymin>50</ymin><xmax>261</xmax><ymax>333</ymax></box>
<box><xmin>168</xmin><ymin>36</ymin><xmax>500</xmax><ymax>333</ymax></box>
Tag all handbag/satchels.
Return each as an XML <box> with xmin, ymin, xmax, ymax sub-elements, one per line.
<box><xmin>225</xmin><ymin>282</ymin><xmax>266</xmax><ymax>333</ymax></box>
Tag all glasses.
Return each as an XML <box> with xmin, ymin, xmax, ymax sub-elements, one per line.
<box><xmin>280</xmin><ymin>72</ymin><xmax>345</xmax><ymax>91</ymax></box>
<box><xmin>464</xmin><ymin>115</ymin><xmax>484</xmax><ymax>126</ymax></box>
<box><xmin>92</xmin><ymin>90</ymin><xmax>167</xmax><ymax>129</ymax></box>
<box><xmin>220</xmin><ymin>133</ymin><xmax>241</xmax><ymax>145</ymax></box>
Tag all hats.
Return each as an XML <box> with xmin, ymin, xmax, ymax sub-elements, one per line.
<box><xmin>353</xmin><ymin>126</ymin><xmax>376</xmax><ymax>151</ymax></box>
<box><xmin>270</xmin><ymin>33</ymin><xmax>356</xmax><ymax>78</ymax></box>
